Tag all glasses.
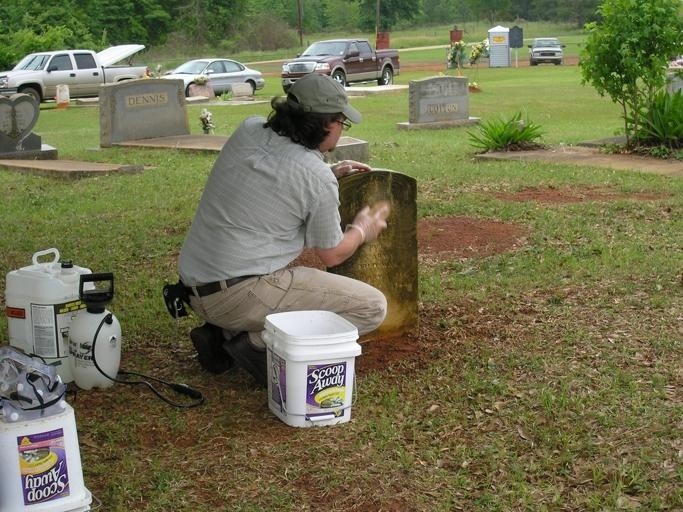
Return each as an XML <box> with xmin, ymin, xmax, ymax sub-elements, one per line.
<box><xmin>334</xmin><ymin>120</ymin><xmax>352</xmax><ymax>132</ymax></box>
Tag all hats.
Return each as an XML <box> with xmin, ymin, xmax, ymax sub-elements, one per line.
<box><xmin>287</xmin><ymin>72</ymin><xmax>364</xmax><ymax>125</ymax></box>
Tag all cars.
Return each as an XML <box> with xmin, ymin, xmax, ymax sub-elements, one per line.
<box><xmin>156</xmin><ymin>58</ymin><xmax>265</xmax><ymax>97</ymax></box>
<box><xmin>528</xmin><ymin>38</ymin><xmax>566</xmax><ymax>65</ymax></box>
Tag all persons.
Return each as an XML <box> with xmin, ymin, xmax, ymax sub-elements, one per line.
<box><xmin>175</xmin><ymin>71</ymin><xmax>392</xmax><ymax>393</ymax></box>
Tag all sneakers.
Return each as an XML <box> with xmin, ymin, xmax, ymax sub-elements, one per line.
<box><xmin>222</xmin><ymin>329</ymin><xmax>268</xmax><ymax>390</ymax></box>
<box><xmin>189</xmin><ymin>321</ymin><xmax>232</xmax><ymax>373</ymax></box>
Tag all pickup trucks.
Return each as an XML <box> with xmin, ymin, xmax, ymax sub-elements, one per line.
<box><xmin>0</xmin><ymin>44</ymin><xmax>156</xmax><ymax>106</ymax></box>
<box><xmin>282</xmin><ymin>38</ymin><xmax>400</xmax><ymax>94</ymax></box>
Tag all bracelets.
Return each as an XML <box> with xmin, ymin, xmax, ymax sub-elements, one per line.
<box><xmin>344</xmin><ymin>221</ymin><xmax>366</xmax><ymax>247</ymax></box>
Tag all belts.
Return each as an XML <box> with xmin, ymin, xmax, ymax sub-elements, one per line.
<box><xmin>177</xmin><ymin>275</ymin><xmax>261</xmax><ymax>297</ymax></box>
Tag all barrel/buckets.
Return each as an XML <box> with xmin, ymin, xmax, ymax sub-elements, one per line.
<box><xmin>4</xmin><ymin>248</ymin><xmax>97</xmax><ymax>385</ymax></box>
<box><xmin>262</xmin><ymin>310</ymin><xmax>363</xmax><ymax>428</ymax></box>
<box><xmin>0</xmin><ymin>394</ymin><xmax>92</xmax><ymax>512</ymax></box>
